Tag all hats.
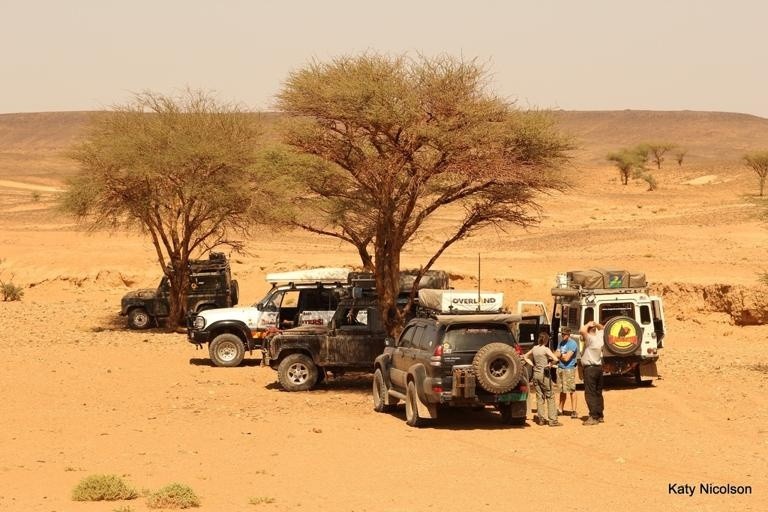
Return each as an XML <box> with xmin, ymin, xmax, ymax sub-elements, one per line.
<box><xmin>557</xmin><ymin>327</ymin><xmax>576</xmax><ymax>334</ymax></box>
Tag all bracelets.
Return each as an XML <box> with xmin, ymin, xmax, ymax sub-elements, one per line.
<box><xmin>558</xmin><ymin>354</ymin><xmax>563</xmax><ymax>360</ymax></box>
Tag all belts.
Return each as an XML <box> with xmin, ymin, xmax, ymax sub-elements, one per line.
<box><xmin>585</xmin><ymin>365</ymin><xmax>600</xmax><ymax>367</ymax></box>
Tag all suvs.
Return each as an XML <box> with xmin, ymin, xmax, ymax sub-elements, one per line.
<box><xmin>372</xmin><ymin>288</ymin><xmax>531</xmax><ymax>427</ymax></box>
<box><xmin>513</xmin><ymin>269</ymin><xmax>668</xmax><ymax>386</ymax></box>
<box><xmin>264</xmin><ymin>271</ymin><xmax>441</xmax><ymax>391</ymax></box>
<box><xmin>119</xmin><ymin>251</ymin><xmax>238</xmax><ymax>329</ymax></box>
<box><xmin>187</xmin><ymin>266</ymin><xmax>351</xmax><ymax>368</ymax></box>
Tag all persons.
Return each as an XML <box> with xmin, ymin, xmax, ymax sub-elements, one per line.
<box><xmin>553</xmin><ymin>326</ymin><xmax>579</xmax><ymax>419</ymax></box>
<box><xmin>522</xmin><ymin>332</ymin><xmax>562</xmax><ymax>427</ymax></box>
<box><xmin>577</xmin><ymin>320</ymin><xmax>604</xmax><ymax>426</ymax></box>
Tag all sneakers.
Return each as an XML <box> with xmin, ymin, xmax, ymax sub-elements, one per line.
<box><xmin>538</xmin><ymin>419</ymin><xmax>546</xmax><ymax>426</ymax></box>
<box><xmin>571</xmin><ymin>410</ymin><xmax>577</xmax><ymax>418</ymax></box>
<box><xmin>548</xmin><ymin>420</ymin><xmax>564</xmax><ymax>426</ymax></box>
<box><xmin>582</xmin><ymin>415</ymin><xmax>604</xmax><ymax>425</ymax></box>
<box><xmin>557</xmin><ymin>409</ymin><xmax>564</xmax><ymax>416</ymax></box>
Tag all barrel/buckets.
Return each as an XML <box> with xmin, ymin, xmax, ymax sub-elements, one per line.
<box><xmin>556</xmin><ymin>273</ymin><xmax>572</xmax><ymax>289</ymax></box>
